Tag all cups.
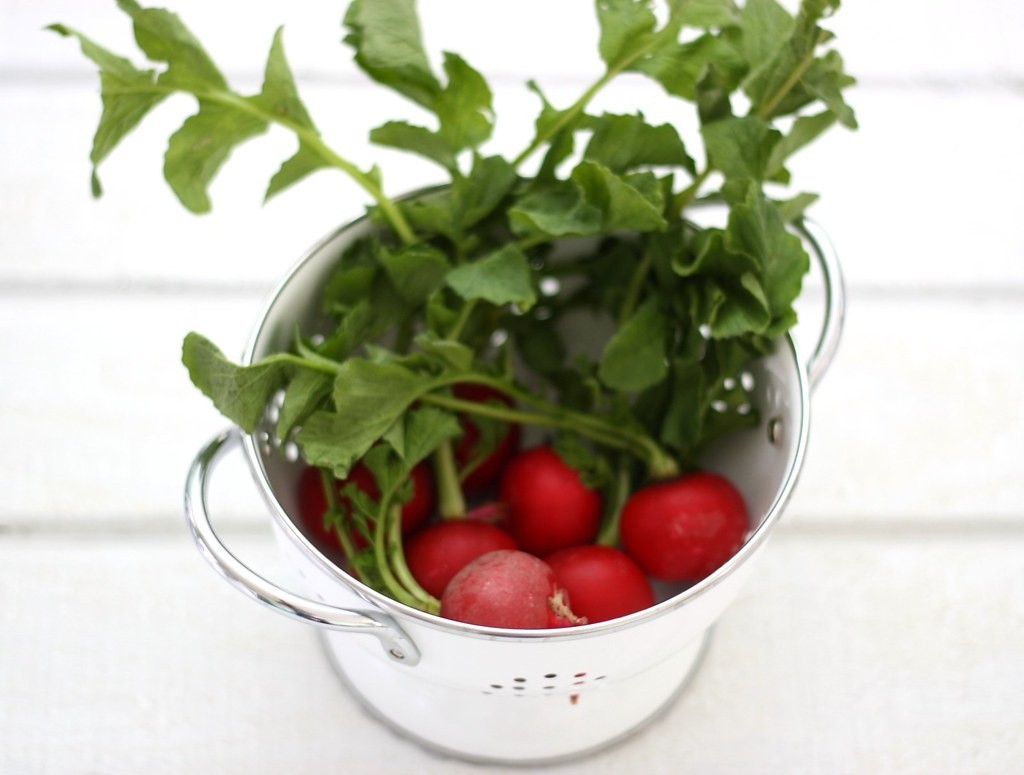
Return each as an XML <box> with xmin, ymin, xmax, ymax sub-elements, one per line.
<box><xmin>182</xmin><ymin>172</ymin><xmax>847</xmax><ymax>766</ymax></box>
